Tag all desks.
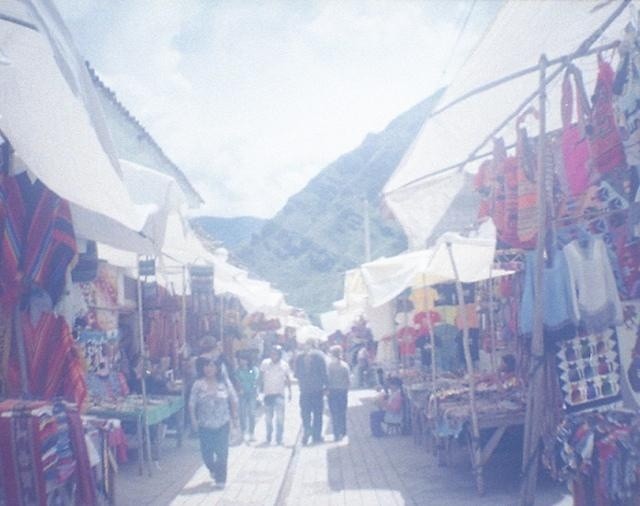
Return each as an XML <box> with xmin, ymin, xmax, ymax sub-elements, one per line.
<box><xmin>92</xmin><ymin>394</ymin><xmax>185</xmax><ymax>477</ymax></box>
<box><xmin>439</xmin><ymin>400</ymin><xmax>527</xmax><ymax>499</ymax></box>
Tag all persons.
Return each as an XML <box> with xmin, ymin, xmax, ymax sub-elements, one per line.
<box><xmin>496</xmin><ymin>355</ymin><xmax>516</xmax><ymax>389</ymax></box>
<box><xmin>189</xmin><ymin>326</ymin><xmax>387</xmax><ymax>447</ymax></box>
<box><xmin>188</xmin><ymin>359</ymin><xmax>239</xmax><ymax>489</ymax></box>
<box><xmin>369</xmin><ymin>376</ymin><xmax>405</xmax><ymax>440</ymax></box>
